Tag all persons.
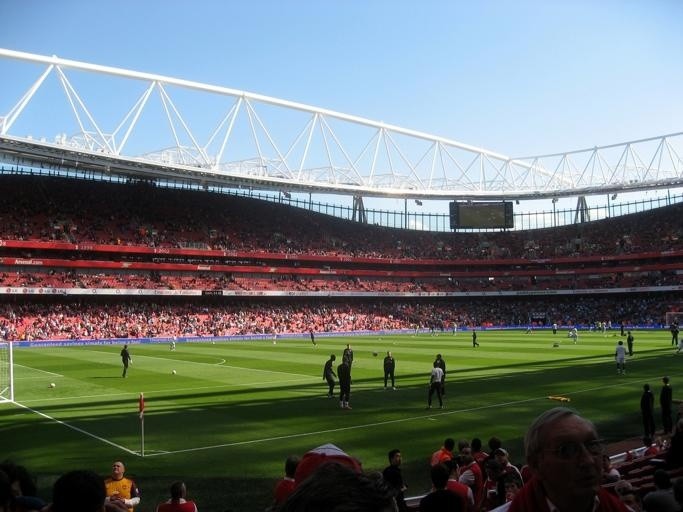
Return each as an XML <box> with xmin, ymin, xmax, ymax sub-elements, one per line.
<box><xmin>613</xmin><ymin>340</ymin><xmax>626</xmax><ymax>375</ymax></box>
<box><xmin>424</xmin><ymin>360</ymin><xmax>443</xmax><ymax>409</ymax></box>
<box><xmin>321</xmin><ymin>354</ymin><xmax>337</xmax><ymax>400</ymax></box>
<box><xmin>431</xmin><ymin>353</ymin><xmax>446</xmax><ymax>398</ymax></box>
<box><xmin>119</xmin><ymin>344</ymin><xmax>131</xmax><ymax>377</ymax></box>
<box><xmin>310</xmin><ymin>331</ymin><xmax>317</xmax><ymax>345</ymax></box>
<box><xmin>671</xmin><ymin>322</ymin><xmax>679</xmax><ymax>346</ymax></box>
<box><xmin>340</xmin><ymin>342</ymin><xmax>354</xmax><ymax>383</ymax></box>
<box><xmin>334</xmin><ymin>356</ymin><xmax>353</xmax><ymax>411</ymax></box>
<box><xmin>638</xmin><ymin>381</ymin><xmax>656</xmax><ymax>447</ymax></box>
<box><xmin>658</xmin><ymin>374</ymin><xmax>673</xmax><ymax>436</ymax></box>
<box><xmin>472</xmin><ymin>328</ymin><xmax>479</xmax><ymax>347</ymax></box>
<box><xmin>1</xmin><ymin>179</ymin><xmax>683</xmax><ymax>342</ymax></box>
<box><xmin>382</xmin><ymin>351</ymin><xmax>397</xmax><ymax>391</ymax></box>
<box><xmin>0</xmin><ymin>407</ymin><xmax>682</xmax><ymax>512</ymax></box>
<box><xmin>627</xmin><ymin>331</ymin><xmax>635</xmax><ymax>356</ymax></box>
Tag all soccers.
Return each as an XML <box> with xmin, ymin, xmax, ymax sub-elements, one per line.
<box><xmin>172</xmin><ymin>370</ymin><xmax>176</xmax><ymax>374</ymax></box>
<box><xmin>49</xmin><ymin>383</ymin><xmax>54</xmax><ymax>387</ymax></box>
<box><xmin>373</xmin><ymin>351</ymin><xmax>378</xmax><ymax>356</ymax></box>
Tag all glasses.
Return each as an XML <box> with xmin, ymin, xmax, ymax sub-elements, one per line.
<box><xmin>544</xmin><ymin>434</ymin><xmax>608</xmax><ymax>462</ymax></box>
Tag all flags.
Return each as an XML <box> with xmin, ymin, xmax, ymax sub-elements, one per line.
<box><xmin>138</xmin><ymin>393</ymin><xmax>145</xmax><ymax>419</ymax></box>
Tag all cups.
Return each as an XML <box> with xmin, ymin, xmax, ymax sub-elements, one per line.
<box><xmin>112</xmin><ymin>491</ymin><xmax>120</xmax><ymax>498</ymax></box>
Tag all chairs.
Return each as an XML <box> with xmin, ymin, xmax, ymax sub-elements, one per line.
<box><xmin>602</xmin><ymin>450</ymin><xmax>682</xmax><ymax>511</ymax></box>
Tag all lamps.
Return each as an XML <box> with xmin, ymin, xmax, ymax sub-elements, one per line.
<box><xmin>280</xmin><ymin>190</ymin><xmax>620</xmax><ymax>207</ymax></box>
